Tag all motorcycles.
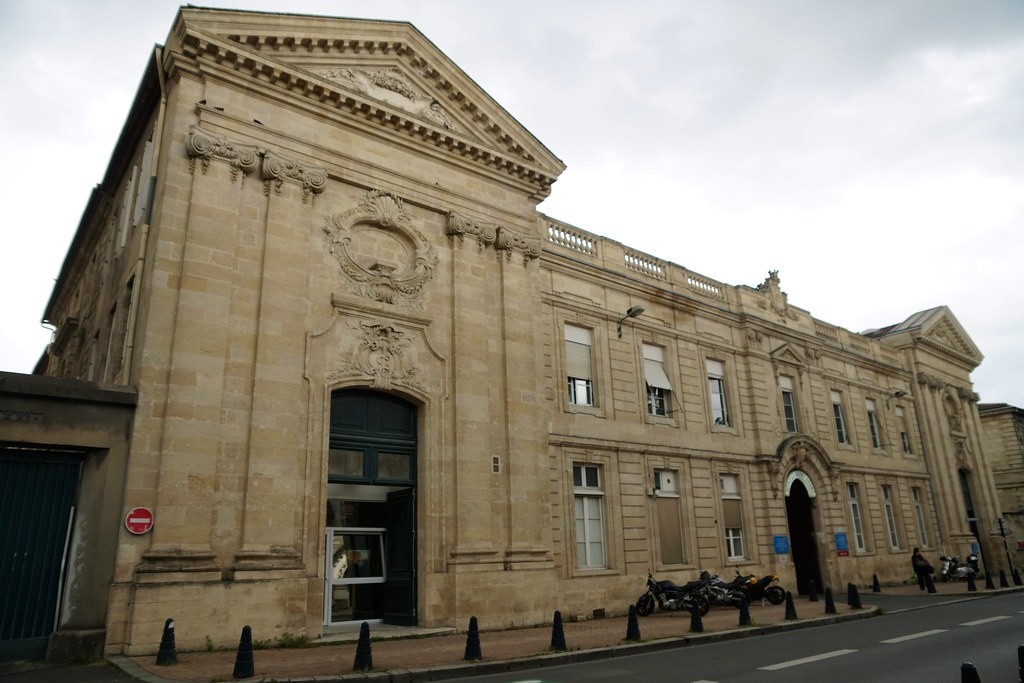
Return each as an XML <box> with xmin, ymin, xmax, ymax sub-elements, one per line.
<box><xmin>634</xmin><ymin>569</ymin><xmax>715</xmax><ymax>617</ymax></box>
<box><xmin>699</xmin><ymin>564</ymin><xmax>787</xmax><ymax>610</ymax></box>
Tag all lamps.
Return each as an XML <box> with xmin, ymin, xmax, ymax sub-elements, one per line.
<box><xmin>617</xmin><ymin>305</ymin><xmax>646</xmax><ymax>339</ymax></box>
<box><xmin>886</xmin><ymin>390</ymin><xmax>908</xmax><ymax>410</ymax></box>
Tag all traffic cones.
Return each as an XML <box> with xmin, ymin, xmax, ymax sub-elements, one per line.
<box><xmin>464</xmin><ymin>616</ymin><xmax>485</xmax><ymax>662</ymax></box>
<box><xmin>999</xmin><ymin>568</ymin><xmax>1010</xmax><ymax>588</ymax></box>
<box><xmin>872</xmin><ymin>574</ymin><xmax>882</xmax><ymax>593</ymax></box>
<box><xmin>156</xmin><ymin>618</ymin><xmax>182</xmax><ymax>666</ymax></box>
<box><xmin>1013</xmin><ymin>567</ymin><xmax>1024</xmax><ymax>586</ymax></box>
<box><xmin>846</xmin><ymin>582</ymin><xmax>864</xmax><ymax>612</ymax></box>
<box><xmin>966</xmin><ymin>572</ymin><xmax>978</xmax><ymax>592</ymax></box>
<box><xmin>687</xmin><ymin>601</ymin><xmax>704</xmax><ymax>634</ymax></box>
<box><xmin>808</xmin><ymin>579</ymin><xmax>819</xmax><ymax>602</ymax></box>
<box><xmin>623</xmin><ymin>603</ymin><xmax>644</xmax><ymax>642</ymax></box>
<box><xmin>232</xmin><ymin>624</ymin><xmax>257</xmax><ymax>680</ymax></box>
<box><xmin>738</xmin><ymin>599</ymin><xmax>754</xmax><ymax>627</ymax></box>
<box><xmin>960</xmin><ymin>662</ymin><xmax>981</xmax><ymax>683</ymax></box>
<box><xmin>548</xmin><ymin>610</ymin><xmax>569</xmax><ymax>653</ymax></box>
<box><xmin>823</xmin><ymin>586</ymin><xmax>836</xmax><ymax>615</ymax></box>
<box><xmin>351</xmin><ymin>622</ymin><xmax>377</xmax><ymax>673</ymax></box>
<box><xmin>984</xmin><ymin>571</ymin><xmax>995</xmax><ymax>590</ymax></box>
<box><xmin>784</xmin><ymin>591</ymin><xmax>799</xmax><ymax>620</ymax></box>
<box><xmin>925</xmin><ymin>573</ymin><xmax>937</xmax><ymax>593</ymax></box>
<box><xmin>1016</xmin><ymin>644</ymin><xmax>1024</xmax><ymax>683</ymax></box>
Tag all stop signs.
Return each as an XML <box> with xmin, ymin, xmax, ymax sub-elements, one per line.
<box><xmin>126</xmin><ymin>507</ymin><xmax>154</xmax><ymax>535</ymax></box>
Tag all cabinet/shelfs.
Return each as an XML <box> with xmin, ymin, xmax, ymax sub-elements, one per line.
<box><xmin>324</xmin><ymin>527</ymin><xmax>387</xmax><ymax>628</ymax></box>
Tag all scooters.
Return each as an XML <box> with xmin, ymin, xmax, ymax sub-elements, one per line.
<box><xmin>940</xmin><ymin>552</ymin><xmax>978</xmax><ymax>581</ymax></box>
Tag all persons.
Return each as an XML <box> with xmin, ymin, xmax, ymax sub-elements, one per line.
<box><xmin>911</xmin><ymin>547</ymin><xmax>933</xmax><ymax>590</ymax></box>
